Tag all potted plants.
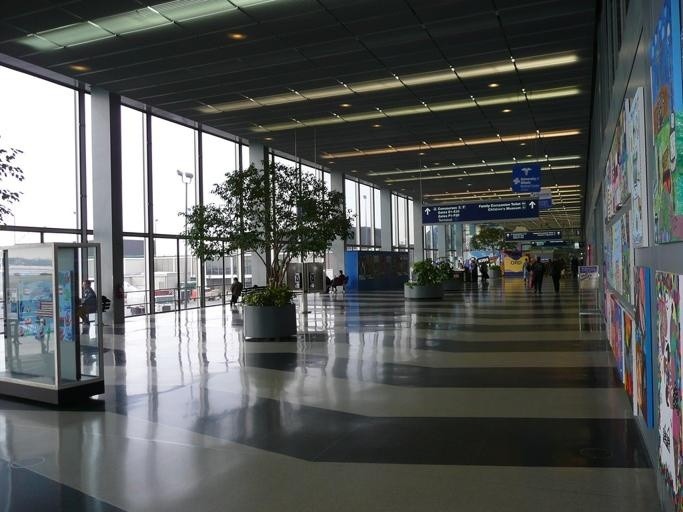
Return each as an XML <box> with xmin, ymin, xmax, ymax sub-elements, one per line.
<box><xmin>471</xmin><ymin>228</ymin><xmax>517</xmax><ymax>279</ymax></box>
<box><xmin>176</xmin><ymin>160</ymin><xmax>357</xmax><ymax>339</ymax></box>
<box><xmin>403</xmin><ymin>258</ymin><xmax>464</xmax><ymax>299</ymax></box>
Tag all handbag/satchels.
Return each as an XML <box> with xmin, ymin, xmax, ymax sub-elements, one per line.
<box><xmin>526</xmin><ymin>265</ymin><xmax>530</xmax><ymax>272</ymax></box>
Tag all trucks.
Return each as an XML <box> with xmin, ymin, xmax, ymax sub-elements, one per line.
<box><xmin>126</xmin><ymin>289</ymin><xmax>174</xmax><ymax>312</ymax></box>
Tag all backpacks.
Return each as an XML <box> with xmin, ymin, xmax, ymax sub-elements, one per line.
<box><xmin>534</xmin><ymin>262</ymin><xmax>542</xmax><ymax>273</ymax></box>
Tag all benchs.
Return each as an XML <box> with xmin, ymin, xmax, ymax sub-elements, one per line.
<box><xmin>33</xmin><ymin>294</ymin><xmax>110</xmax><ymax>332</ymax></box>
<box><xmin>327</xmin><ymin>278</ymin><xmax>348</xmax><ymax>292</ymax></box>
<box><xmin>230</xmin><ymin>286</ymin><xmax>269</xmax><ymax>307</ymax></box>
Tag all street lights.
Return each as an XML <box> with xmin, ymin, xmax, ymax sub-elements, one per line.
<box><xmin>154</xmin><ymin>219</ymin><xmax>159</xmax><ymax>233</ymax></box>
<box><xmin>177</xmin><ymin>170</ymin><xmax>193</xmax><ymax>309</ymax></box>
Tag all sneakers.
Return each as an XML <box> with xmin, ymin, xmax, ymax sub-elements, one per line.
<box><xmin>535</xmin><ymin>291</ymin><xmax>543</xmax><ymax>294</ymax></box>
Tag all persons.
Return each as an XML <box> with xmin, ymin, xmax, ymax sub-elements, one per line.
<box><xmin>331</xmin><ymin>269</ymin><xmax>345</xmax><ymax>287</ymax></box>
<box><xmin>230</xmin><ymin>277</ymin><xmax>243</xmax><ymax>306</ymax></box>
<box><xmin>35</xmin><ymin>315</ymin><xmax>46</xmax><ymax>352</ymax></box>
<box><xmin>80</xmin><ymin>280</ymin><xmax>96</xmax><ymax>325</ymax></box>
<box><xmin>458</xmin><ymin>259</ymin><xmax>490</xmax><ymax>284</ymax></box>
<box><xmin>326</xmin><ymin>277</ymin><xmax>331</xmax><ymax>292</ymax></box>
<box><xmin>521</xmin><ymin>256</ymin><xmax>567</xmax><ymax>294</ymax></box>
